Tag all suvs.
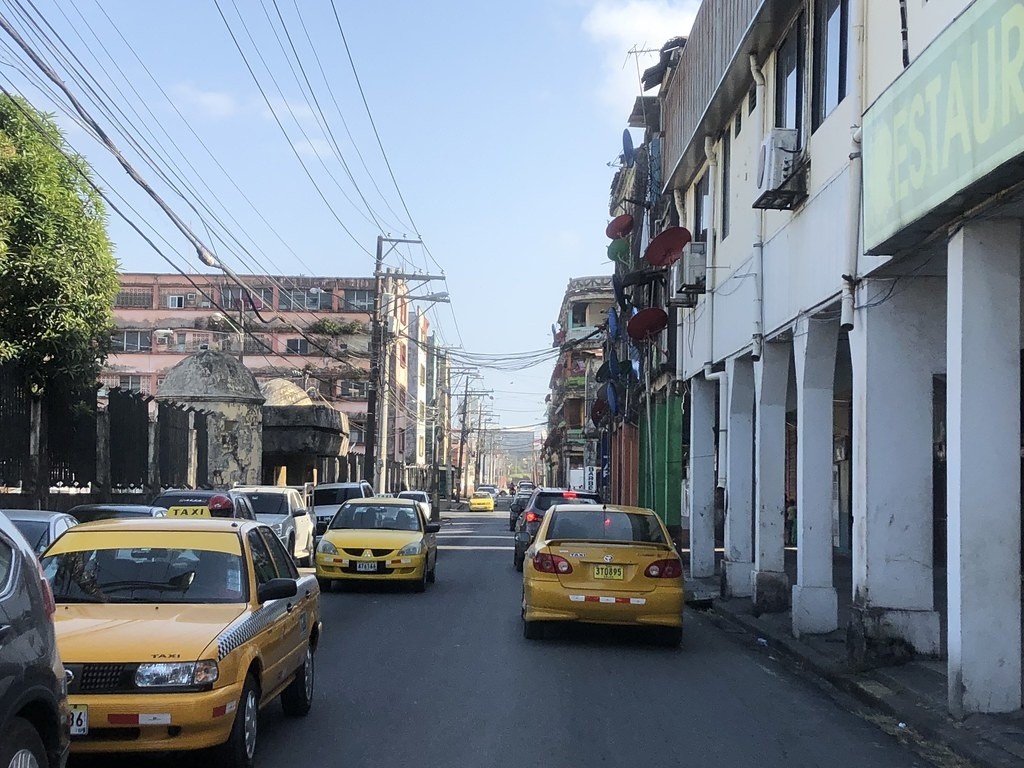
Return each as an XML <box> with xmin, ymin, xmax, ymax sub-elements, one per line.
<box><xmin>514</xmin><ymin>485</ymin><xmax>603</xmax><ymax>571</ymax></box>
<box><xmin>307</xmin><ymin>480</ymin><xmax>376</xmax><ymax>543</ymax></box>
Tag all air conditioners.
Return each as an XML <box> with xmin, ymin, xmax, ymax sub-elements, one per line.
<box><xmin>157</xmin><ymin>337</ymin><xmax>167</xmax><ymax>345</ymax></box>
<box><xmin>669</xmin><ymin>259</ymin><xmax>695</xmax><ymax>307</ymax></box>
<box><xmin>755</xmin><ymin>128</ymin><xmax>799</xmax><ymax>206</ymax></box>
<box><xmin>187</xmin><ymin>294</ymin><xmax>196</xmax><ymax>300</ymax></box>
<box><xmin>158</xmin><ymin>379</ymin><xmax>164</xmax><ymax>385</ymax></box>
<box><xmin>678</xmin><ymin>241</ymin><xmax>706</xmax><ymax>294</ymax></box>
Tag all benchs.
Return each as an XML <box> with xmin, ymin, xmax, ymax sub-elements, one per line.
<box><xmin>108</xmin><ymin>559</ymin><xmax>193</xmax><ymax>591</ymax></box>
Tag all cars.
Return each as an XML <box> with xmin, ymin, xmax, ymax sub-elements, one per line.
<box><xmin>521</xmin><ymin>504</ymin><xmax>686</xmax><ymax>646</ymax></box>
<box><xmin>397</xmin><ymin>490</ymin><xmax>433</xmax><ymax>521</ymax></box>
<box><xmin>0</xmin><ymin>512</ymin><xmax>76</xmax><ymax>768</ymax></box>
<box><xmin>0</xmin><ymin>509</ymin><xmax>81</xmax><ymax>555</ymax></box>
<box><xmin>315</xmin><ymin>497</ymin><xmax>442</xmax><ymax>593</ymax></box>
<box><xmin>476</xmin><ymin>487</ymin><xmax>498</xmax><ymax>506</ymax></box>
<box><xmin>468</xmin><ymin>492</ymin><xmax>495</xmax><ymax>512</ymax></box>
<box><xmin>38</xmin><ymin>516</ymin><xmax>324</xmax><ymax>768</ymax></box>
<box><xmin>148</xmin><ymin>487</ymin><xmax>257</xmax><ymax>521</ymax></box>
<box><xmin>66</xmin><ymin>504</ymin><xmax>168</xmax><ymax>523</ymax></box>
<box><xmin>231</xmin><ymin>486</ymin><xmax>314</xmax><ymax>568</ymax></box>
<box><xmin>509</xmin><ymin>480</ymin><xmax>537</xmax><ymax>531</ymax></box>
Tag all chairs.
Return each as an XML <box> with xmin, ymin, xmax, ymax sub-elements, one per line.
<box><xmin>396</xmin><ymin>510</ymin><xmax>411</xmax><ymax>528</ymax></box>
<box><xmin>184</xmin><ymin>554</ymin><xmax>230</xmax><ymax>599</ymax></box>
<box><xmin>365</xmin><ymin>507</ymin><xmax>377</xmax><ymax>527</ymax></box>
<box><xmin>353</xmin><ymin>512</ymin><xmax>369</xmax><ymax>527</ymax></box>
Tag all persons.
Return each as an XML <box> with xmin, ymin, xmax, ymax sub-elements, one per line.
<box><xmin>198</xmin><ymin>495</ymin><xmax>234</xmax><ymax>566</ymax></box>
<box><xmin>510</xmin><ymin>482</ymin><xmax>515</xmax><ymax>491</ymax></box>
